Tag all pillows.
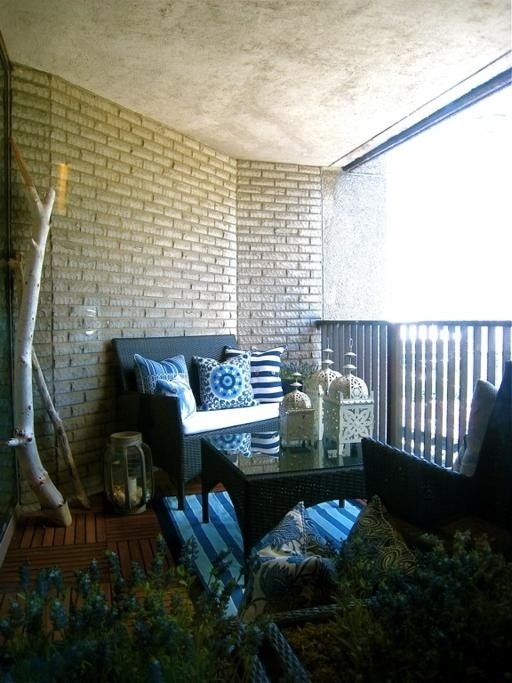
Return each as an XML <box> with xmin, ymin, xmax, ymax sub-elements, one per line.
<box><xmin>340</xmin><ymin>495</ymin><xmax>423</xmax><ymax>596</ymax></box>
<box><xmin>452</xmin><ymin>379</ymin><xmax>498</xmax><ymax>478</ymax></box>
<box><xmin>156</xmin><ymin>375</ymin><xmax>197</xmax><ymax>421</ymax></box>
<box><xmin>133</xmin><ymin>353</ymin><xmax>191</xmax><ymax>394</ymax></box>
<box><xmin>209</xmin><ymin>431</ymin><xmax>280</xmax><ymax>458</ymax></box>
<box><xmin>192</xmin><ymin>350</ymin><xmax>260</xmax><ymax>411</ymax></box>
<box><xmin>224</xmin><ymin>344</ymin><xmax>287</xmax><ymax>403</ymax></box>
<box><xmin>243</xmin><ymin>500</ymin><xmax>343</xmax><ymax>623</ymax></box>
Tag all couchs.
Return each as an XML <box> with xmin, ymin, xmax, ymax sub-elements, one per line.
<box><xmin>110</xmin><ymin>334</ymin><xmax>284</xmax><ymax>510</ymax></box>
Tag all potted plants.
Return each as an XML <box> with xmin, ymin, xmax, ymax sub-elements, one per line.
<box><xmin>0</xmin><ymin>535</ymin><xmax>271</xmax><ymax>683</ymax></box>
<box><xmin>265</xmin><ymin>529</ymin><xmax>512</xmax><ymax>683</ymax></box>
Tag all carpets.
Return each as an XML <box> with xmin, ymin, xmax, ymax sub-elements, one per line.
<box><xmin>157</xmin><ymin>488</ymin><xmax>368</xmax><ymax>631</ymax></box>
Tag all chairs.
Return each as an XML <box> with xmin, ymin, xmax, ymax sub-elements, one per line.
<box><xmin>362</xmin><ymin>360</ymin><xmax>512</xmax><ymax>532</ymax></box>
